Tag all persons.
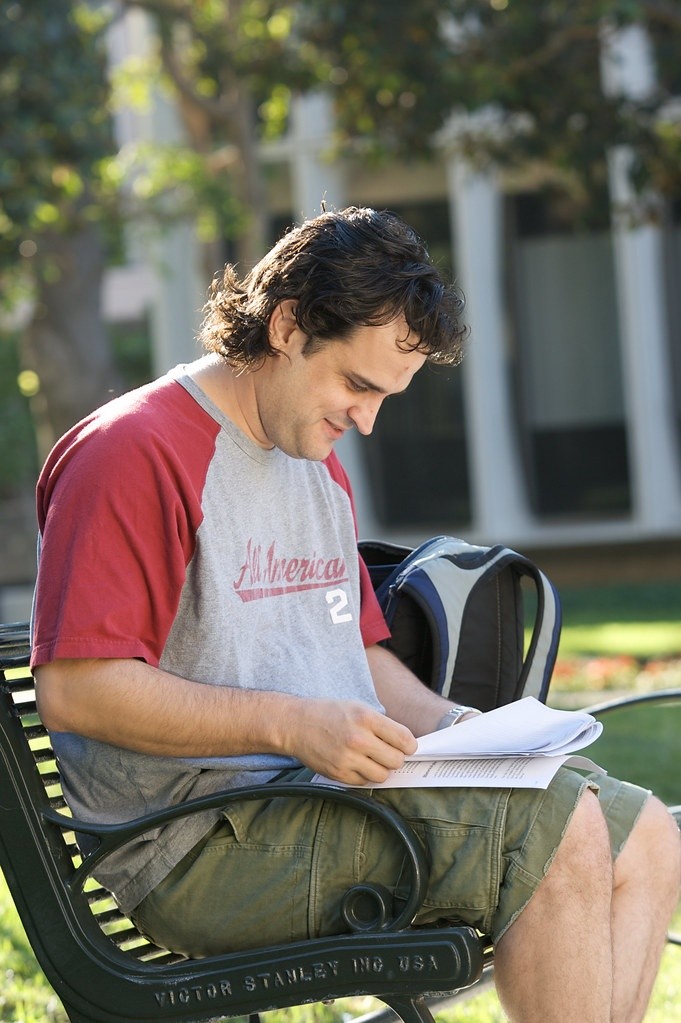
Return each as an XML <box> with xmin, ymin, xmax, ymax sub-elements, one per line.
<box><xmin>28</xmin><ymin>205</ymin><xmax>681</xmax><ymax>1023</ymax></box>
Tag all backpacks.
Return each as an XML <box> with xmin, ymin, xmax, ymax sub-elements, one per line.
<box><xmin>357</xmin><ymin>535</ymin><xmax>562</xmax><ymax>714</ymax></box>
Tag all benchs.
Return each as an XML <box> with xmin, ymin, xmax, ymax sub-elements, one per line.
<box><xmin>0</xmin><ymin>562</ymin><xmax>681</xmax><ymax>1022</ymax></box>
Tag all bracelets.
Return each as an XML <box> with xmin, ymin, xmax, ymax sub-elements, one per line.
<box><xmin>438</xmin><ymin>706</ymin><xmax>482</xmax><ymax>732</ymax></box>
